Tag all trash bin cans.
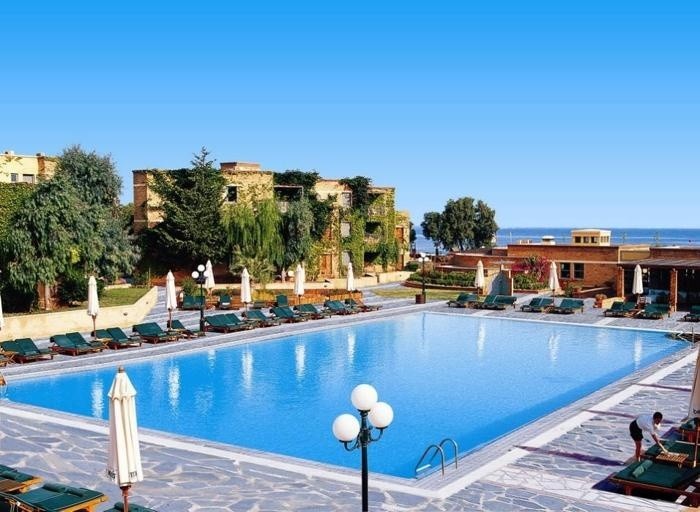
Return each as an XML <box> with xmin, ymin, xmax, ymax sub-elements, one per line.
<box><xmin>415</xmin><ymin>294</ymin><xmax>425</xmax><ymax>304</ymax></box>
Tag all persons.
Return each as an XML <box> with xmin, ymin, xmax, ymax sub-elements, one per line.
<box><xmin>629</xmin><ymin>412</ymin><xmax>669</xmax><ymax>461</ymax></box>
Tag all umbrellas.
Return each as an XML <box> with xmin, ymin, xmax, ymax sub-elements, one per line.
<box><xmin>294</xmin><ymin>264</ymin><xmax>305</xmax><ymax>305</ymax></box>
<box><xmin>548</xmin><ymin>261</ymin><xmax>561</xmax><ymax>303</ymax></box>
<box><xmin>293</xmin><ymin>336</ymin><xmax>308</xmax><ymax>380</ymax></box>
<box><xmin>346</xmin><ymin>262</ymin><xmax>355</xmax><ymax>305</ymax></box>
<box><xmin>345</xmin><ymin>326</ymin><xmax>356</xmax><ymax>365</ymax></box>
<box><xmin>473</xmin><ymin>260</ymin><xmax>485</xmax><ymax>298</ymax></box>
<box><xmin>631</xmin><ymin>330</ymin><xmax>646</xmax><ymax>373</ymax></box>
<box><xmin>240</xmin><ymin>267</ymin><xmax>252</xmax><ymax>312</ymax></box>
<box><xmin>549</xmin><ymin>324</ymin><xmax>561</xmax><ymax>364</ymax></box>
<box><xmin>687</xmin><ymin>346</ymin><xmax>700</xmax><ymax>467</ymax></box>
<box><xmin>632</xmin><ymin>264</ymin><xmax>644</xmax><ymax>305</ymax></box>
<box><xmin>89</xmin><ymin>366</ymin><xmax>104</xmax><ymax>420</ymax></box>
<box><xmin>165</xmin><ymin>269</ymin><xmax>177</xmax><ymax>328</ymax></box>
<box><xmin>206</xmin><ymin>258</ymin><xmax>216</xmax><ymax>304</ymax></box>
<box><xmin>240</xmin><ymin>342</ymin><xmax>255</xmax><ymax>397</ymax></box>
<box><xmin>107</xmin><ymin>365</ymin><xmax>144</xmax><ymax>511</ymax></box>
<box><xmin>166</xmin><ymin>355</ymin><xmax>181</xmax><ymax>410</ymax></box>
<box><xmin>475</xmin><ymin>317</ymin><xmax>487</xmax><ymax>358</ymax></box>
<box><xmin>87</xmin><ymin>275</ymin><xmax>100</xmax><ymax>339</ymax></box>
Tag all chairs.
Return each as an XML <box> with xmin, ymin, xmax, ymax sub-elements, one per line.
<box><xmin>0</xmin><ymin>317</ymin><xmax>202</xmax><ymax>369</ymax></box>
<box><xmin>0</xmin><ymin>465</ymin><xmax>156</xmax><ymax>511</ymax></box>
<box><xmin>447</xmin><ymin>291</ymin><xmax>699</xmax><ymax>322</ymax></box>
<box><xmin>177</xmin><ymin>294</ymin><xmax>383</xmax><ymax>334</ymax></box>
<box><xmin>611</xmin><ymin>418</ymin><xmax>700</xmax><ymax>504</ymax></box>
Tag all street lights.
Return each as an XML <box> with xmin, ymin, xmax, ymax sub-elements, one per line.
<box><xmin>191</xmin><ymin>264</ymin><xmax>209</xmax><ymax>332</ymax></box>
<box><xmin>418</xmin><ymin>251</ymin><xmax>431</xmax><ymax>304</ymax></box>
<box><xmin>332</xmin><ymin>382</ymin><xmax>393</xmax><ymax>512</ymax></box>
